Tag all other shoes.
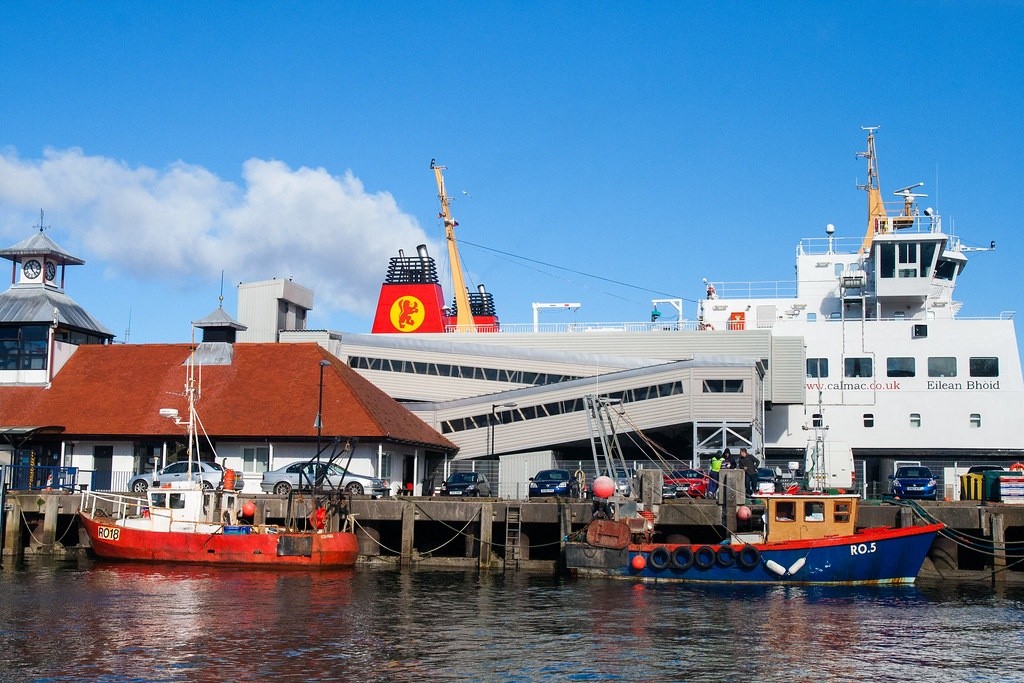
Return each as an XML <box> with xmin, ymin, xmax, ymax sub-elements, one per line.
<box><xmin>708</xmin><ymin>491</ymin><xmax>714</xmax><ymax>497</ymax></box>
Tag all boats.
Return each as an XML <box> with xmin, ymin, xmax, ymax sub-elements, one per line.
<box><xmin>556</xmin><ymin>388</ymin><xmax>948</xmax><ymax>587</ymax></box>
<box><xmin>75</xmin><ymin>319</ymin><xmax>360</xmax><ymax>569</ymax></box>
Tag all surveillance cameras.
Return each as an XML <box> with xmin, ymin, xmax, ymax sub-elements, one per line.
<box><xmin>159</xmin><ymin>409</ymin><xmax>178</xmax><ymax>419</ymax></box>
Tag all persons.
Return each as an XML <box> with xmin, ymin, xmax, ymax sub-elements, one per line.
<box><xmin>708</xmin><ymin>448</ymin><xmax>760</xmax><ymax>497</ymax></box>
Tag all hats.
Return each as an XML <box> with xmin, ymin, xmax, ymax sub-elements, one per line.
<box><xmin>725</xmin><ymin>448</ymin><xmax>731</xmax><ymax>453</ymax></box>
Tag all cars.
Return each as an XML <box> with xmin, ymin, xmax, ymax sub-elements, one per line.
<box><xmin>439</xmin><ymin>471</ymin><xmax>493</xmax><ymax>499</ymax></box>
<box><xmin>959</xmin><ymin>464</ymin><xmax>1004</xmax><ymax>500</ymax></box>
<box><xmin>661</xmin><ymin>467</ymin><xmax>710</xmax><ymax>500</ymax></box>
<box><xmin>661</xmin><ymin>485</ymin><xmax>677</xmax><ymax>498</ymax></box>
<box><xmin>528</xmin><ymin>469</ymin><xmax>580</xmax><ymax>499</ymax></box>
<box><xmin>592</xmin><ymin>466</ymin><xmax>640</xmax><ymax>497</ymax></box>
<box><xmin>750</xmin><ymin>468</ymin><xmax>785</xmax><ymax>495</ymax></box>
<box><xmin>260</xmin><ymin>460</ymin><xmax>391</xmax><ymax>497</ymax></box>
<box><xmin>888</xmin><ymin>465</ymin><xmax>941</xmax><ymax>500</ymax></box>
<box><xmin>127</xmin><ymin>461</ymin><xmax>245</xmax><ymax>491</ymax></box>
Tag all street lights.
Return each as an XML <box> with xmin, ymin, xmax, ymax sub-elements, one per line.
<box><xmin>492</xmin><ymin>402</ymin><xmax>518</xmax><ymax>461</ymax></box>
<box><xmin>314</xmin><ymin>359</ymin><xmax>330</xmax><ymax>488</ymax></box>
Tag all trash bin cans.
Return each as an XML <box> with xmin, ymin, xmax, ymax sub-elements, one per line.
<box><xmin>729</xmin><ymin>311</ymin><xmax>746</xmax><ymax>330</ymax></box>
<box><xmin>960</xmin><ymin>472</ymin><xmax>983</xmax><ymax>499</ymax></box>
